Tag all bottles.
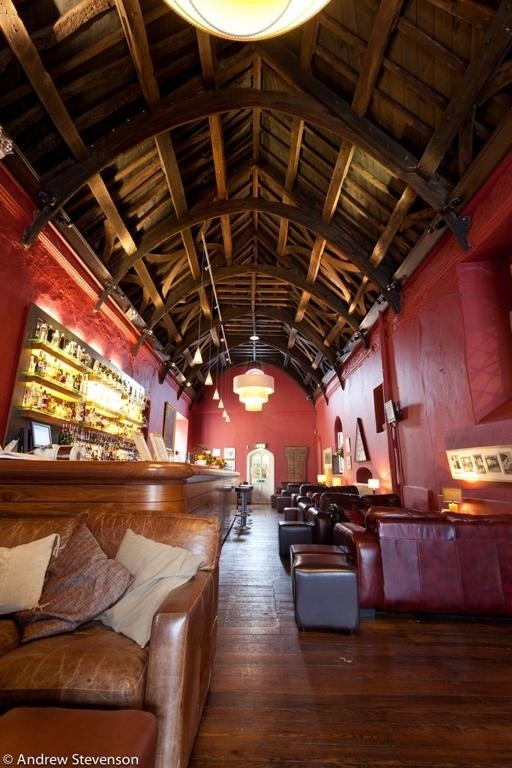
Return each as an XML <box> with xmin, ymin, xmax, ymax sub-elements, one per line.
<box><xmin>22</xmin><ymin>323</ymin><xmax>136</xmax><ymax>461</ymax></box>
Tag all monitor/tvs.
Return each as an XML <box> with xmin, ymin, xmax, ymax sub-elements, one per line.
<box><xmin>384</xmin><ymin>399</ymin><xmax>396</xmax><ymax>424</ymax></box>
<box><xmin>32</xmin><ymin>422</ymin><xmax>52</xmax><ymax>448</ymax></box>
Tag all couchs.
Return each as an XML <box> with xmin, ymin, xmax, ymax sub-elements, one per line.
<box><xmin>0</xmin><ymin>511</ymin><xmax>220</xmax><ymax>768</ymax></box>
<box><xmin>288</xmin><ymin>481</ymin><xmax>512</xmax><ymax>612</ymax></box>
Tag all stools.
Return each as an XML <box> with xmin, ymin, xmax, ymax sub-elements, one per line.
<box><xmin>234</xmin><ymin>484</ymin><xmax>254</xmax><ymax>531</ymax></box>
<box><xmin>2</xmin><ymin>705</ymin><xmax>160</xmax><ymax>767</ymax></box>
<box><xmin>271</xmin><ymin>493</ymin><xmax>359</xmax><ymax>632</ymax></box>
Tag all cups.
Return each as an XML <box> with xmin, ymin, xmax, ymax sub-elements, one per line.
<box><xmin>195</xmin><ymin>460</ymin><xmax>206</xmax><ymax>465</ymax></box>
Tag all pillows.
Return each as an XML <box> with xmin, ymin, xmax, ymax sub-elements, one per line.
<box><xmin>1</xmin><ymin>524</ymin><xmax>203</xmax><ymax>650</ymax></box>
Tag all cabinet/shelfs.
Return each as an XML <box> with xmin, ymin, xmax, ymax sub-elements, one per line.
<box><xmin>4</xmin><ymin>301</ymin><xmax>149</xmax><ymax>455</ymax></box>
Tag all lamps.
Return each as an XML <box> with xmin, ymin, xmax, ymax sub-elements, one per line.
<box><xmin>191</xmin><ymin>243</ymin><xmax>276</xmax><ymax>414</ymax></box>
